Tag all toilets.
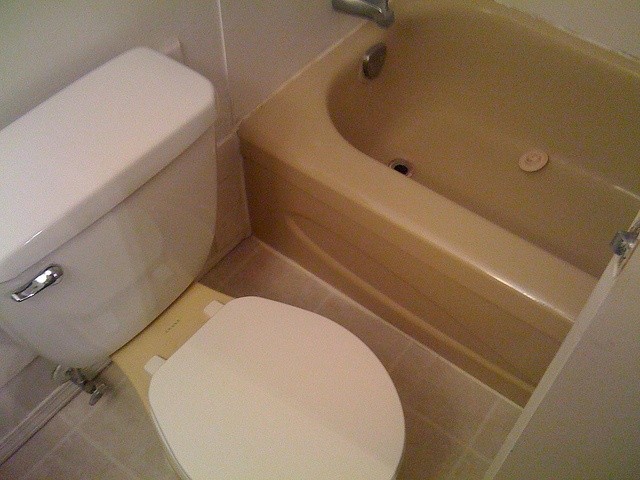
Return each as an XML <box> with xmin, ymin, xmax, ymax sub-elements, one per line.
<box><xmin>0</xmin><ymin>45</ymin><xmax>407</xmax><ymax>480</ymax></box>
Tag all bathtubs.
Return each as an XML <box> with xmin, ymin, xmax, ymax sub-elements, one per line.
<box><xmin>235</xmin><ymin>1</ymin><xmax>640</xmax><ymax>391</ymax></box>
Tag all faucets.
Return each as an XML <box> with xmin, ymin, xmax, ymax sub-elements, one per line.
<box><xmin>332</xmin><ymin>0</ymin><xmax>395</xmax><ymax>29</ymax></box>
